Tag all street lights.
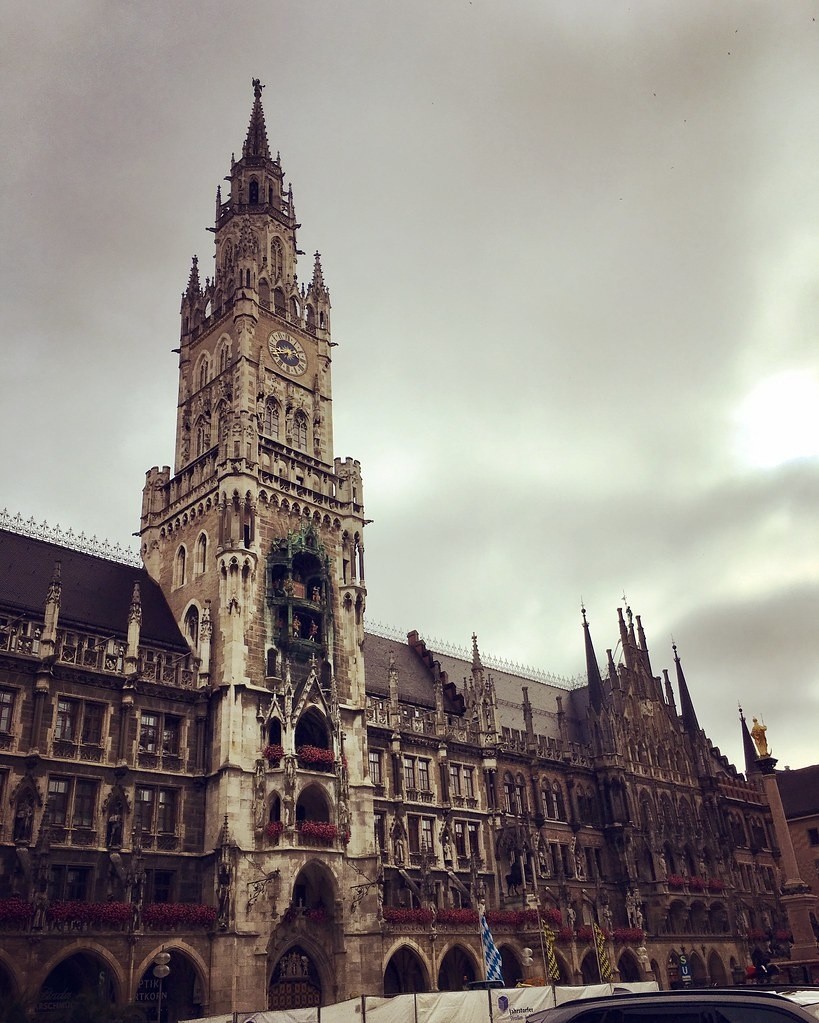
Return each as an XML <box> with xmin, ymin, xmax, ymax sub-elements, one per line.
<box><xmin>521</xmin><ymin>947</ymin><xmax>534</xmax><ymax>984</ymax></box>
<box><xmin>153</xmin><ymin>944</ymin><xmax>170</xmax><ymax>1023</ymax></box>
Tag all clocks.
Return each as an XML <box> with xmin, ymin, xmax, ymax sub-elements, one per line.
<box><xmin>266</xmin><ymin>329</ymin><xmax>309</xmax><ymax>377</ymax></box>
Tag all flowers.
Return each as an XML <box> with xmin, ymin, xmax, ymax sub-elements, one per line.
<box><xmin>266</xmin><ymin>819</ymin><xmax>351</xmax><ymax>844</ymax></box>
<box><xmin>377</xmin><ymin>901</ymin><xmax>645</xmax><ymax>943</ymax></box>
<box><xmin>668</xmin><ymin>873</ymin><xmax>725</xmax><ymax>891</ymax></box>
<box><xmin>745</xmin><ymin>927</ymin><xmax>792</xmax><ymax>939</ymax></box>
<box><xmin>258</xmin><ymin>743</ymin><xmax>336</xmax><ymax>766</ymax></box>
<box><xmin>0</xmin><ymin>890</ymin><xmax>223</xmax><ymax>930</ymax></box>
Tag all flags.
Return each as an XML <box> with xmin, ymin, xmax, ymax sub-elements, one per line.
<box><xmin>481</xmin><ymin>917</ymin><xmax>502</xmax><ymax>980</ymax></box>
<box><xmin>541</xmin><ymin>919</ymin><xmax>560</xmax><ymax>981</ymax></box>
<box><xmin>594</xmin><ymin>923</ymin><xmax>614</xmax><ymax>981</ymax></box>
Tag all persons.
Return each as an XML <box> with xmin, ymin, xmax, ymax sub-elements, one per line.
<box><xmin>659</xmin><ymin>853</ymin><xmax>667</xmax><ymax>880</ymax></box>
<box><xmin>479</xmin><ymin>880</ymin><xmax>485</xmax><ymax>895</ymax></box>
<box><xmin>478</xmin><ymin>899</ymin><xmax>486</xmax><ymax>924</ymax></box>
<box><xmin>577</xmin><ymin>857</ymin><xmax>581</xmax><ymax>878</ymax></box>
<box><xmin>603</xmin><ymin>905</ymin><xmax>613</xmax><ymax>933</ymax></box>
<box><xmin>699</xmin><ymin>859</ymin><xmax>707</xmax><ymax>881</ymax></box>
<box><xmin>680</xmin><ymin>856</ymin><xmax>688</xmax><ymax>877</ymax></box>
<box><xmin>293</xmin><ymin>616</ymin><xmax>301</xmax><ymax>637</ymax></box>
<box><xmin>566</xmin><ymin>903</ymin><xmax>576</xmax><ymax>930</ymax></box>
<box><xmin>539</xmin><ymin>852</ymin><xmax>547</xmax><ymax>873</ymax></box>
<box><xmin>395</xmin><ymin>836</ymin><xmax>403</xmax><ymax>861</ymax></box>
<box><xmin>444</xmin><ymin>841</ymin><xmax>452</xmax><ymax>866</ymax></box>
<box><xmin>751</xmin><ymin>719</ymin><xmax>767</xmax><ymax>755</ymax></box>
<box><xmin>428</xmin><ymin>899</ymin><xmax>436</xmax><ymax>930</ymax></box>
<box><xmin>637</xmin><ymin>908</ymin><xmax>643</xmax><ymax>929</ymax></box>
<box><xmin>378</xmin><ymin>884</ymin><xmax>387</xmax><ymax>921</ymax></box>
<box><xmin>309</xmin><ymin>620</ymin><xmax>318</xmax><ymax>642</ymax></box>
